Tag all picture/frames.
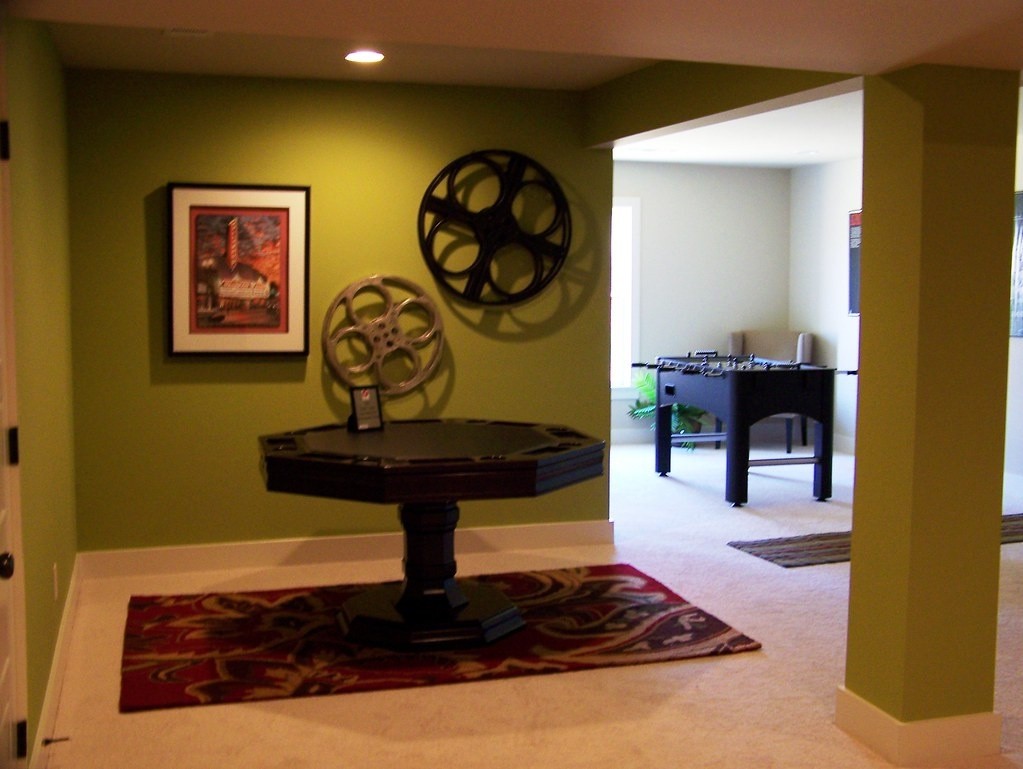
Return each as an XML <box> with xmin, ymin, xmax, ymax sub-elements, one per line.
<box><xmin>167</xmin><ymin>181</ymin><xmax>311</xmax><ymax>358</ymax></box>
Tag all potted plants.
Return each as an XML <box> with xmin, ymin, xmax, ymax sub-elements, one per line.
<box><xmin>626</xmin><ymin>371</ymin><xmax>710</xmax><ymax>452</ymax></box>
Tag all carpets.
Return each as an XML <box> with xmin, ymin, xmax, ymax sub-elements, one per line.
<box><xmin>117</xmin><ymin>563</ymin><xmax>761</xmax><ymax>711</ymax></box>
<box><xmin>727</xmin><ymin>513</ymin><xmax>1023</xmax><ymax>567</ymax></box>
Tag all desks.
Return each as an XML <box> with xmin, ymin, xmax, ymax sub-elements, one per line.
<box><xmin>632</xmin><ymin>350</ymin><xmax>858</xmax><ymax>508</ymax></box>
<box><xmin>257</xmin><ymin>420</ymin><xmax>606</xmax><ymax>651</ymax></box>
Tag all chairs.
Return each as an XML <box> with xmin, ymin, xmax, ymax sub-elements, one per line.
<box><xmin>716</xmin><ymin>331</ymin><xmax>814</xmax><ymax>453</ymax></box>
